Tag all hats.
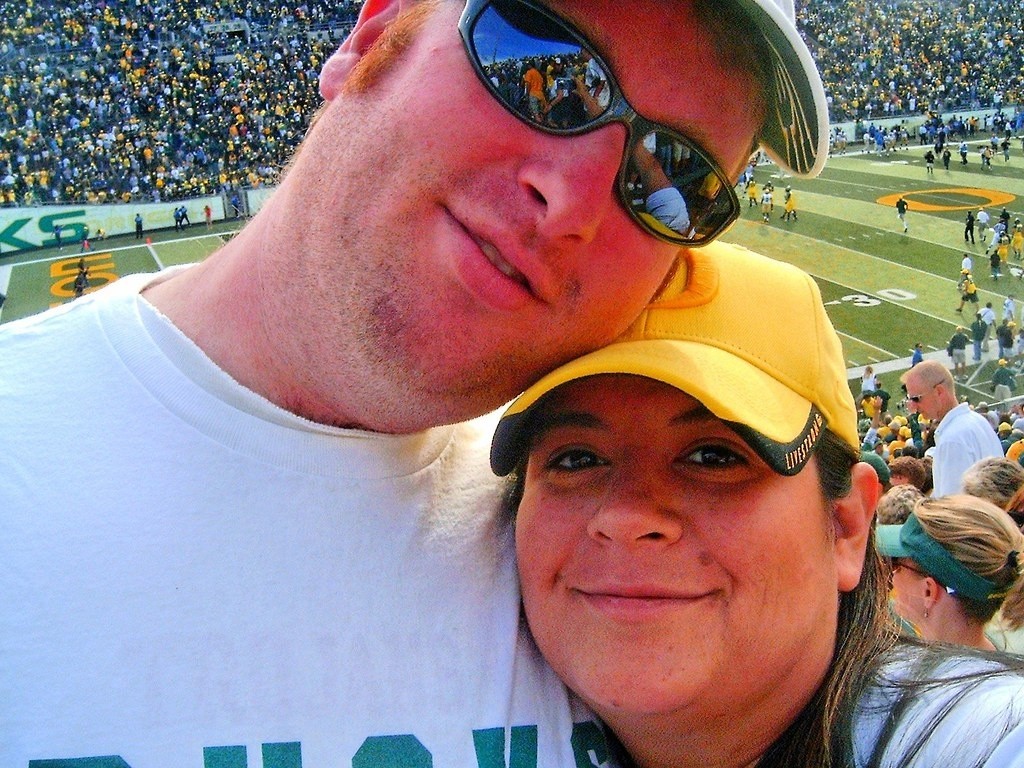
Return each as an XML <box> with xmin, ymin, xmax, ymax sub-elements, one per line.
<box><xmin>998</xmin><ymin>358</ymin><xmax>1008</xmax><ymax>365</ymax></box>
<box><xmin>874</xmin><ymin>514</ymin><xmax>994</xmax><ymax>600</ymax></box>
<box><xmin>1007</xmin><ymin>321</ymin><xmax>1016</xmax><ymax>328</ymax></box>
<box><xmin>487</xmin><ymin>242</ymin><xmax>859</xmax><ymax>479</ymax></box>
<box><xmin>995</xmin><ymin>422</ymin><xmax>1011</xmax><ymax>436</ymax></box>
<box><xmin>959</xmin><ymin>269</ymin><xmax>969</xmax><ymax>274</ymax></box>
<box><xmin>749</xmin><ymin>181</ymin><xmax>755</xmax><ymax>185</ymax></box>
<box><xmin>875</xmin><ymin>412</ymin><xmax>932</xmax><ymax>453</ymax></box>
<box><xmin>711</xmin><ymin>0</ymin><xmax>835</xmax><ymax>183</ymax></box>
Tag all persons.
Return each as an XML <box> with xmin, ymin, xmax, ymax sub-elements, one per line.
<box><xmin>0</xmin><ymin>0</ymin><xmax>828</xmax><ymax>768</ymax></box>
<box><xmin>477</xmin><ymin>49</ymin><xmax>609</xmax><ymax>128</ymax></box>
<box><xmin>72</xmin><ymin>257</ymin><xmax>90</xmax><ymax>298</ymax></box>
<box><xmin>733</xmin><ymin>0</ymin><xmax>1024</xmax><ymax>654</ymax></box>
<box><xmin>490</xmin><ymin>241</ymin><xmax>1024</xmax><ymax>768</ymax></box>
<box><xmin>625</xmin><ymin>132</ymin><xmax>730</xmax><ymax>241</ymax></box>
<box><xmin>0</xmin><ymin>0</ymin><xmax>366</xmax><ymax>253</ymax></box>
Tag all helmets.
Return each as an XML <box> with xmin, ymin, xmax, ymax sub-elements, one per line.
<box><xmin>765</xmin><ymin>180</ymin><xmax>792</xmax><ymax>194</ymax></box>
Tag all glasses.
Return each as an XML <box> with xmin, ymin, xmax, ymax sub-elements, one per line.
<box><xmin>903</xmin><ymin>379</ymin><xmax>945</xmax><ymax>403</ymax></box>
<box><xmin>890</xmin><ymin>556</ymin><xmax>928</xmax><ymax>579</ymax></box>
<box><xmin>458</xmin><ymin>0</ymin><xmax>744</xmax><ymax>249</ymax></box>
